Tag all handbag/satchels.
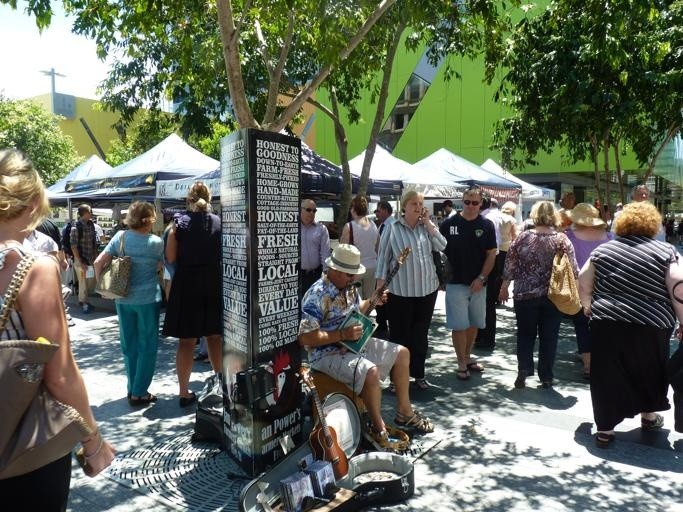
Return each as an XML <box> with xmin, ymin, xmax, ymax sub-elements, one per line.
<box><xmin>432</xmin><ymin>251</ymin><xmax>453</xmax><ymax>284</ymax></box>
<box><xmin>95</xmin><ymin>256</ymin><xmax>133</xmax><ymax>298</ymax></box>
<box><xmin>548</xmin><ymin>252</ymin><xmax>583</xmax><ymax>315</ymax></box>
<box><xmin>61</xmin><ymin>223</ymin><xmax>83</xmax><ymax>256</ymax></box>
<box><xmin>0</xmin><ymin>339</ymin><xmax>92</xmax><ymax>479</ymax></box>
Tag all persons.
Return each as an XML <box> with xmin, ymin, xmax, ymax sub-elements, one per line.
<box><xmin>440</xmin><ymin>189</ymin><xmax>496</xmax><ymax>379</ymax></box>
<box><xmin>157</xmin><ymin>213</ymin><xmax>183</xmax><ymax>301</ymax></box>
<box><xmin>191</xmin><ymin>336</ymin><xmax>211</xmax><ymax>363</ymax></box>
<box><xmin>339</xmin><ymin>196</ymin><xmax>395</xmax><ymax>299</ymax></box>
<box><xmin>23</xmin><ymin>227</ymin><xmax>61</xmax><ymax>269</ymax></box>
<box><xmin>296</xmin><ymin>243</ymin><xmax>433</xmax><ymax>454</ymax></box>
<box><xmin>92</xmin><ymin>198</ymin><xmax>167</xmax><ymax>404</ymax></box>
<box><xmin>498</xmin><ymin>200</ymin><xmax>580</xmax><ymax>387</ymax></box>
<box><xmin>61</xmin><ymin>220</ymin><xmax>78</xmax><ymax>298</ymax></box>
<box><xmin>299</xmin><ymin>199</ymin><xmax>329</xmax><ymax>297</ymax></box>
<box><xmin>71</xmin><ymin>205</ymin><xmax>105</xmax><ymax>315</ymax></box>
<box><xmin>160</xmin><ymin>180</ymin><xmax>224</xmax><ymax>408</ymax></box>
<box><xmin>0</xmin><ymin>145</ymin><xmax>118</xmax><ymax>512</ymax></box>
<box><xmin>574</xmin><ymin>201</ymin><xmax>683</xmax><ymax>448</ymax></box>
<box><xmin>376</xmin><ymin>189</ymin><xmax>447</xmax><ymax>394</ymax></box>
<box><xmin>35</xmin><ymin>216</ymin><xmax>78</xmax><ymax>327</ymax></box>
<box><xmin>443</xmin><ymin>197</ymin><xmax>518</xmax><ymax>304</ymax></box>
<box><xmin>559</xmin><ymin>185</ymin><xmax>682</xmax><ymax>377</ymax></box>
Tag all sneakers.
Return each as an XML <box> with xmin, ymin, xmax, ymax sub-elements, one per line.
<box><xmin>516</xmin><ymin>370</ymin><xmax>527</xmax><ymax>389</ymax></box>
<box><xmin>544</xmin><ymin>376</ymin><xmax>560</xmax><ymax>387</ymax></box>
<box><xmin>415</xmin><ymin>378</ymin><xmax>432</xmax><ymax>390</ymax></box>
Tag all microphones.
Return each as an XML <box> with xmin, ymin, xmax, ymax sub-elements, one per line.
<box><xmin>350</xmin><ymin>282</ymin><xmax>362</xmax><ymax>288</ymax></box>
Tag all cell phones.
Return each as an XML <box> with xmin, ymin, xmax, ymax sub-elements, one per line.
<box><xmin>422</xmin><ymin>207</ymin><xmax>425</xmax><ymax>216</ymax></box>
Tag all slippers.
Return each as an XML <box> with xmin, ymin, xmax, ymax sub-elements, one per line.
<box><xmin>467</xmin><ymin>360</ymin><xmax>484</xmax><ymax>370</ymax></box>
<box><xmin>457</xmin><ymin>369</ymin><xmax>471</xmax><ymax>379</ymax></box>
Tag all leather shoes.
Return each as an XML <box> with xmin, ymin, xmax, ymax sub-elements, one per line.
<box><xmin>179</xmin><ymin>391</ymin><xmax>196</xmax><ymax>406</ymax></box>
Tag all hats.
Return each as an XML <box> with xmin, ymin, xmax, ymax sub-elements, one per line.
<box><xmin>565</xmin><ymin>203</ymin><xmax>608</xmax><ymax>229</ymax></box>
<box><xmin>324</xmin><ymin>245</ymin><xmax>366</xmax><ymax>275</ymax></box>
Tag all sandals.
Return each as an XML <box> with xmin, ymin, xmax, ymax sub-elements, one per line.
<box><xmin>597</xmin><ymin>432</ymin><xmax>614</xmax><ymax>447</ymax></box>
<box><xmin>642</xmin><ymin>414</ymin><xmax>665</xmax><ymax>431</ymax></box>
<box><xmin>395</xmin><ymin>411</ymin><xmax>434</xmax><ymax>433</ymax></box>
<box><xmin>128</xmin><ymin>393</ymin><xmax>157</xmax><ymax>406</ymax></box>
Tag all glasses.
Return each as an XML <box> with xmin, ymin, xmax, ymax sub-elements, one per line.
<box><xmin>463</xmin><ymin>200</ymin><xmax>481</xmax><ymax>205</ymax></box>
<box><xmin>301</xmin><ymin>206</ymin><xmax>317</xmax><ymax>213</ymax></box>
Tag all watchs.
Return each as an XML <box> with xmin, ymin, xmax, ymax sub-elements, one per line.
<box><xmin>478</xmin><ymin>274</ymin><xmax>487</xmax><ymax>286</ymax></box>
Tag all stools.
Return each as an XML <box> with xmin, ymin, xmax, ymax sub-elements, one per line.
<box><xmin>300</xmin><ymin>365</ymin><xmax>381</xmax><ymax>427</ymax></box>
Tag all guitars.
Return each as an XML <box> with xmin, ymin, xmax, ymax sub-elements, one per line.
<box><xmin>295</xmin><ymin>367</ymin><xmax>349</xmax><ymax>483</ymax></box>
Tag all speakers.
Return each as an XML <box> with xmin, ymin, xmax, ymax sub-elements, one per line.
<box><xmin>237</xmin><ymin>360</ymin><xmax>278</xmax><ymax>402</ymax></box>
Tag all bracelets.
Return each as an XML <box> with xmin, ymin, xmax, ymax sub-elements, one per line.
<box><xmin>79</xmin><ymin>430</ymin><xmax>99</xmax><ymax>443</ymax></box>
<box><xmin>80</xmin><ymin>438</ymin><xmax>105</xmax><ymax>468</ymax></box>
